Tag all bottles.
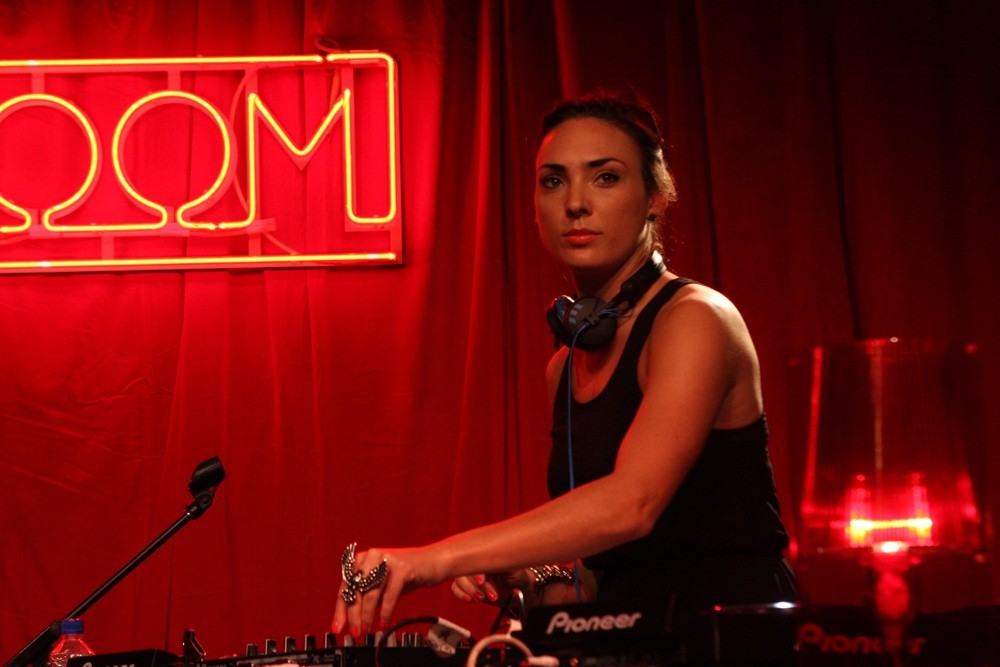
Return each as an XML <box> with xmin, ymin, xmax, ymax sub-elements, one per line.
<box><xmin>47</xmin><ymin>618</ymin><xmax>97</xmax><ymax>667</ymax></box>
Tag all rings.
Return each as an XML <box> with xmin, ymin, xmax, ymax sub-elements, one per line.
<box><xmin>338</xmin><ymin>541</ymin><xmax>388</xmax><ymax>605</ymax></box>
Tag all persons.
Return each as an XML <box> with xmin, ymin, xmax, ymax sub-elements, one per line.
<box><xmin>327</xmin><ymin>91</ymin><xmax>801</xmax><ymax>637</ymax></box>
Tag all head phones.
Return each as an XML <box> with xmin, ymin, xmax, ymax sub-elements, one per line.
<box><xmin>546</xmin><ymin>249</ymin><xmax>668</xmax><ymax>352</ymax></box>
